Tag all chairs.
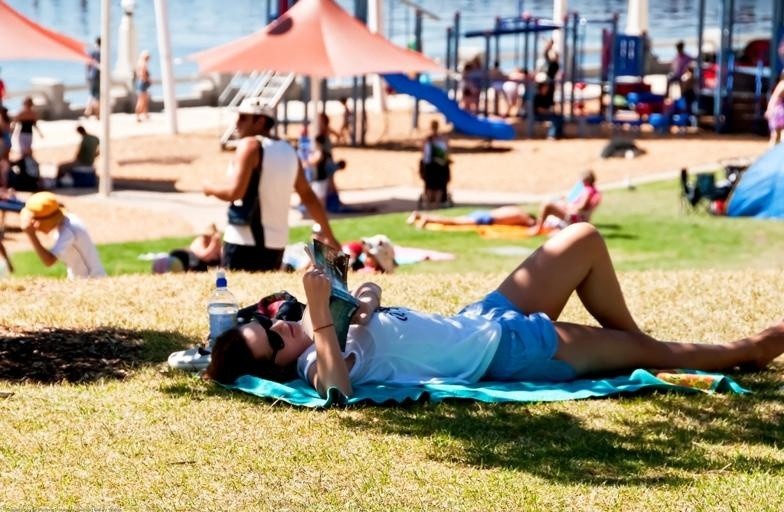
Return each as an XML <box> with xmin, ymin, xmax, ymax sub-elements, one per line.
<box><xmin>556</xmin><ymin>192</ymin><xmax>603</xmax><ymax>235</ymax></box>
<box><xmin>677</xmin><ymin>168</ymin><xmax>709</xmax><ymax>215</ymax></box>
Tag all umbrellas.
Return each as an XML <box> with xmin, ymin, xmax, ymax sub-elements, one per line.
<box><xmin>188</xmin><ymin>0</ymin><xmax>452</xmax><ymax>135</ymax></box>
<box><xmin>1</xmin><ymin>1</ymin><xmax>88</xmax><ymax>62</ymax></box>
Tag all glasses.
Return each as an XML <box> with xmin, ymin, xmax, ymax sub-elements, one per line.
<box><xmin>250</xmin><ymin>312</ymin><xmax>285</xmax><ymax>364</ymax></box>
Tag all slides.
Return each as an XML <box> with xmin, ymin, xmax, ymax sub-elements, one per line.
<box><xmin>382</xmin><ymin>74</ymin><xmax>515</xmax><ymax>139</ymax></box>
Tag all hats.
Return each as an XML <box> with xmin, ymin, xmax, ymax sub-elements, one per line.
<box><xmin>360</xmin><ymin>233</ymin><xmax>396</xmax><ymax>274</ymax></box>
<box><xmin>19</xmin><ymin>191</ymin><xmax>67</xmax><ymax>234</ymax></box>
<box><xmin>228</xmin><ymin>96</ymin><xmax>276</xmax><ymax>121</ymax></box>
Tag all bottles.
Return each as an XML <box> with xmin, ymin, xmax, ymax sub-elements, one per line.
<box><xmin>205</xmin><ymin>272</ymin><xmax>240</xmax><ymax>348</ymax></box>
